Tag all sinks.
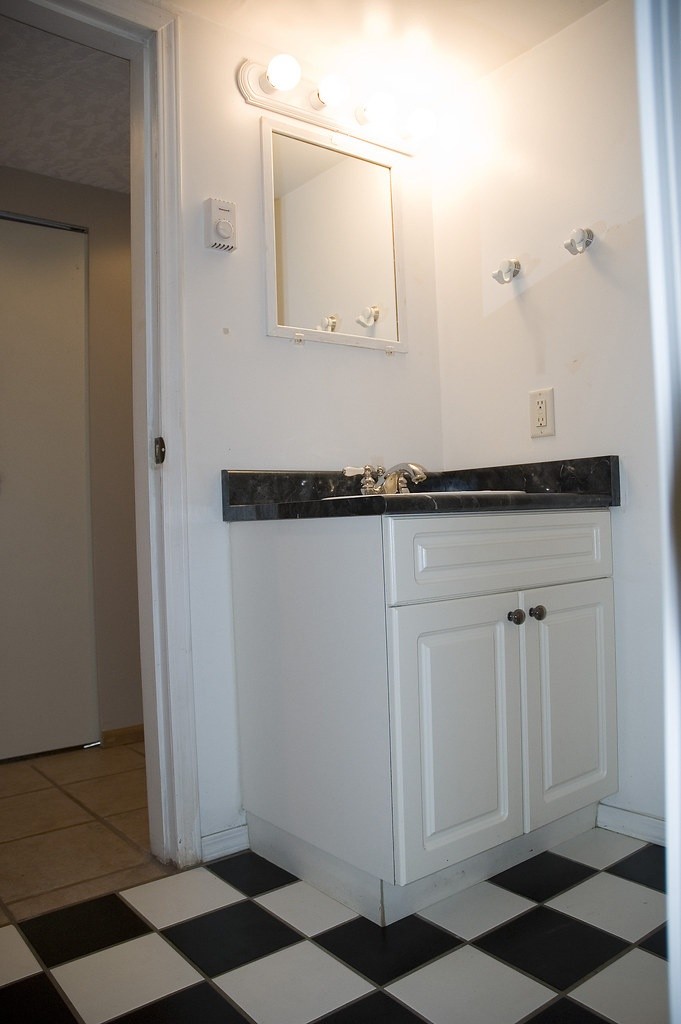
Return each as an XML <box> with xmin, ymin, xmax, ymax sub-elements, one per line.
<box><xmin>320</xmin><ymin>485</ymin><xmax>527</xmax><ymax>501</ymax></box>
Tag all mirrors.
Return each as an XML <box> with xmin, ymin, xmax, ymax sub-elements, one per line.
<box><xmin>261</xmin><ymin>115</ymin><xmax>411</xmax><ymax>356</ymax></box>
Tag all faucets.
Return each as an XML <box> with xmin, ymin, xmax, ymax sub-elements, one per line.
<box><xmin>373</xmin><ymin>463</ymin><xmax>427</xmax><ymax>496</ymax></box>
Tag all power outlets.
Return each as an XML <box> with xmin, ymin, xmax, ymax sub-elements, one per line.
<box><xmin>528</xmin><ymin>387</ymin><xmax>555</xmax><ymax>438</ymax></box>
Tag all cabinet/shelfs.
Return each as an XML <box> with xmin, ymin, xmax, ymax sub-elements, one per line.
<box><xmin>230</xmin><ymin>506</ymin><xmax>620</xmax><ymax>928</ymax></box>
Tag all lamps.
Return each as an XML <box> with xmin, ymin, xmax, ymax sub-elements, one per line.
<box><xmin>237</xmin><ymin>52</ymin><xmax>415</xmax><ymax>155</ymax></box>
